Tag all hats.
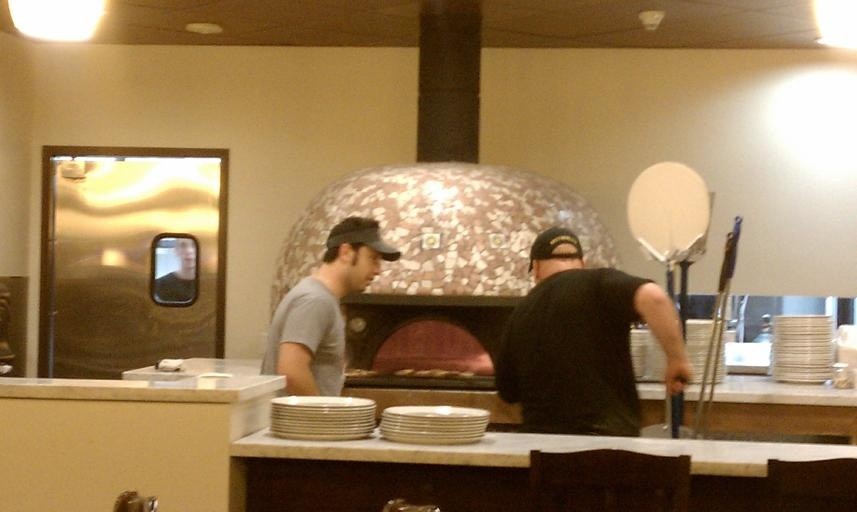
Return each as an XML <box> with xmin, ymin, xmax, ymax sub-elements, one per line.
<box><xmin>326</xmin><ymin>217</ymin><xmax>401</xmax><ymax>262</ymax></box>
<box><xmin>529</xmin><ymin>225</ymin><xmax>582</xmax><ymax>271</ymax></box>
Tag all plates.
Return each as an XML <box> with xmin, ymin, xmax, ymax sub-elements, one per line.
<box><xmin>628</xmin><ymin>319</ymin><xmax>727</xmax><ymax>386</ymax></box>
<box><xmin>769</xmin><ymin>314</ymin><xmax>834</xmax><ymax>385</ymax></box>
<box><xmin>268</xmin><ymin>396</ymin><xmax>491</xmax><ymax>444</ymax></box>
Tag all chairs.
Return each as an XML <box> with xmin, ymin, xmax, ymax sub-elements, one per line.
<box><xmin>768</xmin><ymin>458</ymin><xmax>857</xmax><ymax>510</ymax></box>
<box><xmin>526</xmin><ymin>450</ymin><xmax>694</xmax><ymax>512</ymax></box>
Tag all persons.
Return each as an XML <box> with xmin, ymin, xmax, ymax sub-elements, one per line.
<box><xmin>261</xmin><ymin>217</ymin><xmax>401</xmax><ymax>399</ymax></box>
<box><xmin>490</xmin><ymin>227</ymin><xmax>692</xmax><ymax>438</ymax></box>
<box><xmin>152</xmin><ymin>235</ymin><xmax>197</xmax><ymax>302</ymax></box>
